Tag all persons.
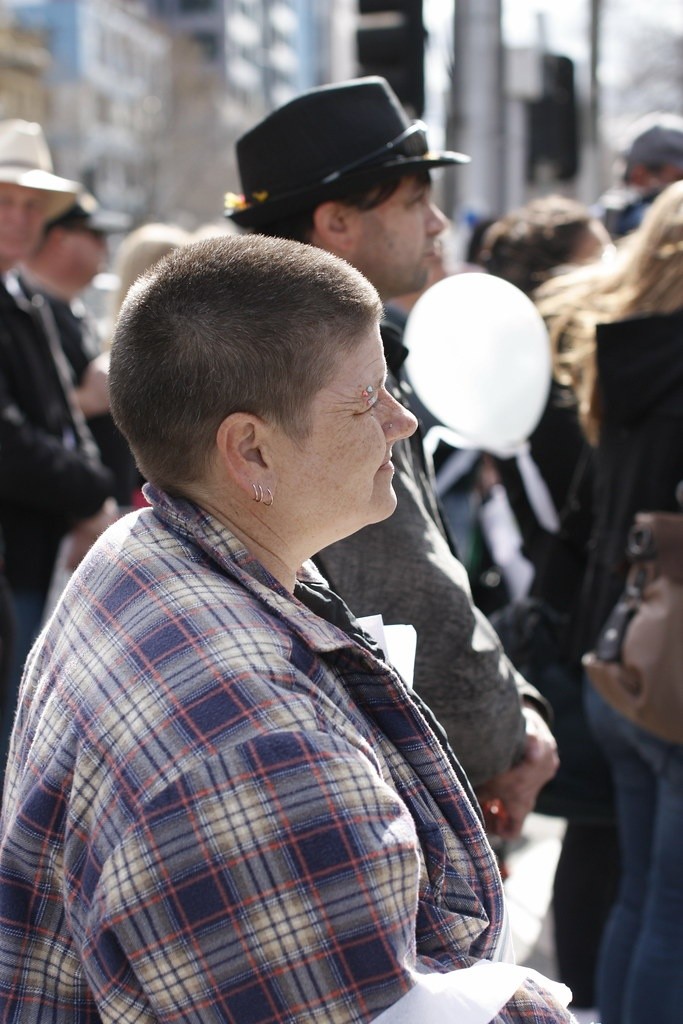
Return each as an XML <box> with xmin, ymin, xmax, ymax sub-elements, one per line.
<box><xmin>0</xmin><ymin>73</ymin><xmax>682</xmax><ymax>1024</ymax></box>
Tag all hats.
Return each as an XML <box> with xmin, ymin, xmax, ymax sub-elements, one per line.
<box><xmin>225</xmin><ymin>76</ymin><xmax>470</xmax><ymax>226</ymax></box>
<box><xmin>46</xmin><ymin>193</ymin><xmax>133</xmax><ymax>232</ymax></box>
<box><xmin>0</xmin><ymin>118</ymin><xmax>82</xmax><ymax>192</ymax></box>
<box><xmin>628</xmin><ymin>126</ymin><xmax>683</xmax><ymax>166</ymax></box>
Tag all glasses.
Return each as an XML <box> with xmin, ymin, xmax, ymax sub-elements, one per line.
<box><xmin>320</xmin><ymin>118</ymin><xmax>430</xmax><ymax>179</ymax></box>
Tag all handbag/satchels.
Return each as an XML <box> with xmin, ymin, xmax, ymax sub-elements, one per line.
<box><xmin>581</xmin><ymin>512</ymin><xmax>683</xmax><ymax>748</ymax></box>
